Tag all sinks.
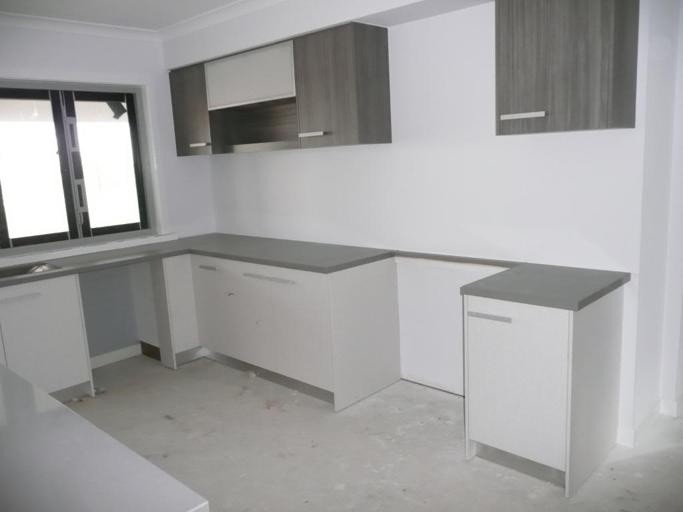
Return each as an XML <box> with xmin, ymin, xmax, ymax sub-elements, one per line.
<box><xmin>0</xmin><ymin>263</ymin><xmax>63</xmax><ymax>279</ymax></box>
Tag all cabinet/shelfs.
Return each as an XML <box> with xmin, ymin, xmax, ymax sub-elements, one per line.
<box><xmin>495</xmin><ymin>1</ymin><xmax>640</xmax><ymax>136</ymax></box>
<box><xmin>462</xmin><ymin>285</ymin><xmax>623</xmax><ymax>499</ymax></box>
<box><xmin>1</xmin><ymin>273</ymin><xmax>95</xmax><ymax>404</ymax></box>
<box><xmin>168</xmin><ymin>21</ymin><xmax>392</xmax><ymax>157</ymax></box>
<box><xmin>191</xmin><ymin>253</ymin><xmax>401</xmax><ymax>412</ymax></box>
